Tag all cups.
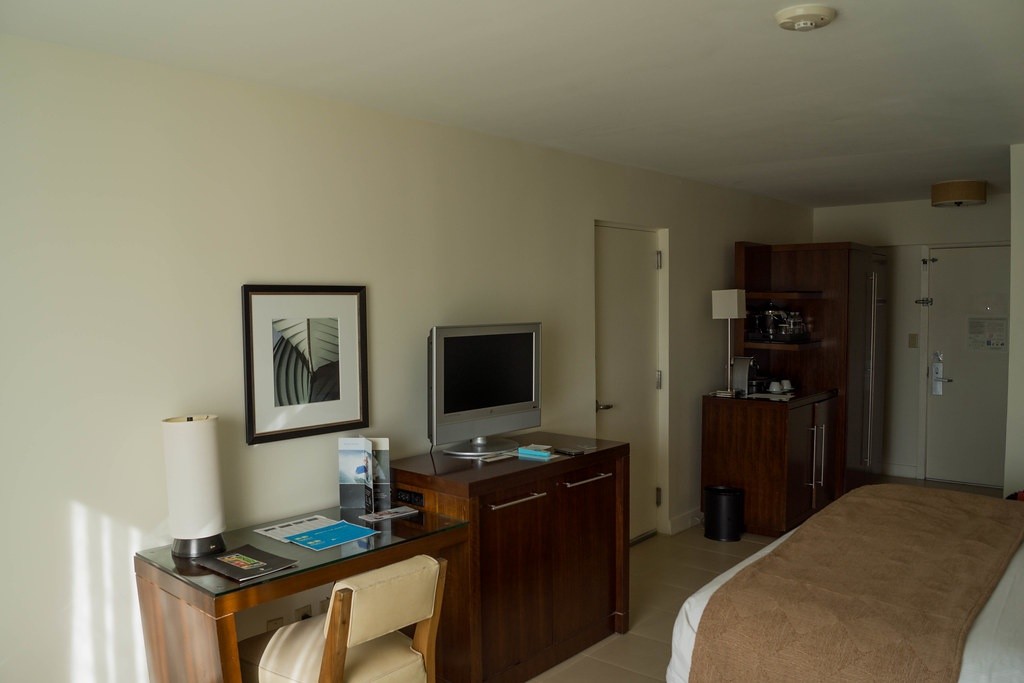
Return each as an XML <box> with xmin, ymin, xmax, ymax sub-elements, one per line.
<box><xmin>781</xmin><ymin>380</ymin><xmax>791</xmax><ymax>389</ymax></box>
<box><xmin>769</xmin><ymin>382</ymin><xmax>782</xmax><ymax>391</ymax></box>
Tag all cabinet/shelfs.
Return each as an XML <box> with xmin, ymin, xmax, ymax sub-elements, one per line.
<box><xmin>701</xmin><ymin>242</ymin><xmax>890</xmax><ymax>538</ymax></box>
<box><xmin>389</xmin><ymin>432</ymin><xmax>629</xmax><ymax>683</ymax></box>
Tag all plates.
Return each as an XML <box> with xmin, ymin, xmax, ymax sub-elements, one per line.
<box><xmin>767</xmin><ymin>390</ymin><xmax>785</xmax><ymax>393</ymax></box>
<box><xmin>782</xmin><ymin>388</ymin><xmax>794</xmax><ymax>392</ymax></box>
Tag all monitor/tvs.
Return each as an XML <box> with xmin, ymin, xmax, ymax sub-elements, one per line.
<box><xmin>426</xmin><ymin>322</ymin><xmax>542</xmax><ymax>456</ymax></box>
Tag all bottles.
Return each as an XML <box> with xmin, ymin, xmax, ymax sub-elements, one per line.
<box><xmin>787</xmin><ymin>312</ymin><xmax>802</xmax><ymax>334</ymax></box>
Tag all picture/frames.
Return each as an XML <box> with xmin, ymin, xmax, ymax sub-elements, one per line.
<box><xmin>241</xmin><ymin>284</ymin><xmax>369</xmax><ymax>446</ymax></box>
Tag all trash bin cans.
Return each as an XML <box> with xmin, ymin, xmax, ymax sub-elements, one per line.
<box><xmin>704</xmin><ymin>485</ymin><xmax>744</xmax><ymax>542</ymax></box>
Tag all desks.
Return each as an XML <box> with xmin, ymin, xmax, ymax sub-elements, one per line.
<box><xmin>134</xmin><ymin>501</ymin><xmax>468</xmax><ymax>683</ymax></box>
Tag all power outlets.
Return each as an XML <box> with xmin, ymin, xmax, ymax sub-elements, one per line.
<box><xmin>295</xmin><ymin>605</ymin><xmax>312</xmax><ymax>622</ymax></box>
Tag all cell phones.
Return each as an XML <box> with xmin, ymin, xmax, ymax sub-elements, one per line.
<box><xmin>556</xmin><ymin>448</ymin><xmax>585</xmax><ymax>455</ymax></box>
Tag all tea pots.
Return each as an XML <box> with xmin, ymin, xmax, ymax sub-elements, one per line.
<box><xmin>754</xmin><ymin>301</ymin><xmax>788</xmax><ymax>336</ymax></box>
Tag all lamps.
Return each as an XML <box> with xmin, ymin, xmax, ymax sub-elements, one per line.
<box><xmin>162</xmin><ymin>414</ymin><xmax>227</xmax><ymax>558</ymax></box>
<box><xmin>930</xmin><ymin>180</ymin><xmax>987</xmax><ymax>208</ymax></box>
<box><xmin>711</xmin><ymin>289</ymin><xmax>747</xmax><ymax>397</ymax></box>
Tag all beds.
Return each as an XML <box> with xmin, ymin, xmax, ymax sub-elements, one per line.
<box><xmin>666</xmin><ymin>482</ymin><xmax>1024</xmax><ymax>683</ymax></box>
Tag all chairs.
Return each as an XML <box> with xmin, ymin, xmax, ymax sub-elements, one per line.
<box><xmin>237</xmin><ymin>554</ymin><xmax>448</xmax><ymax>682</ymax></box>
